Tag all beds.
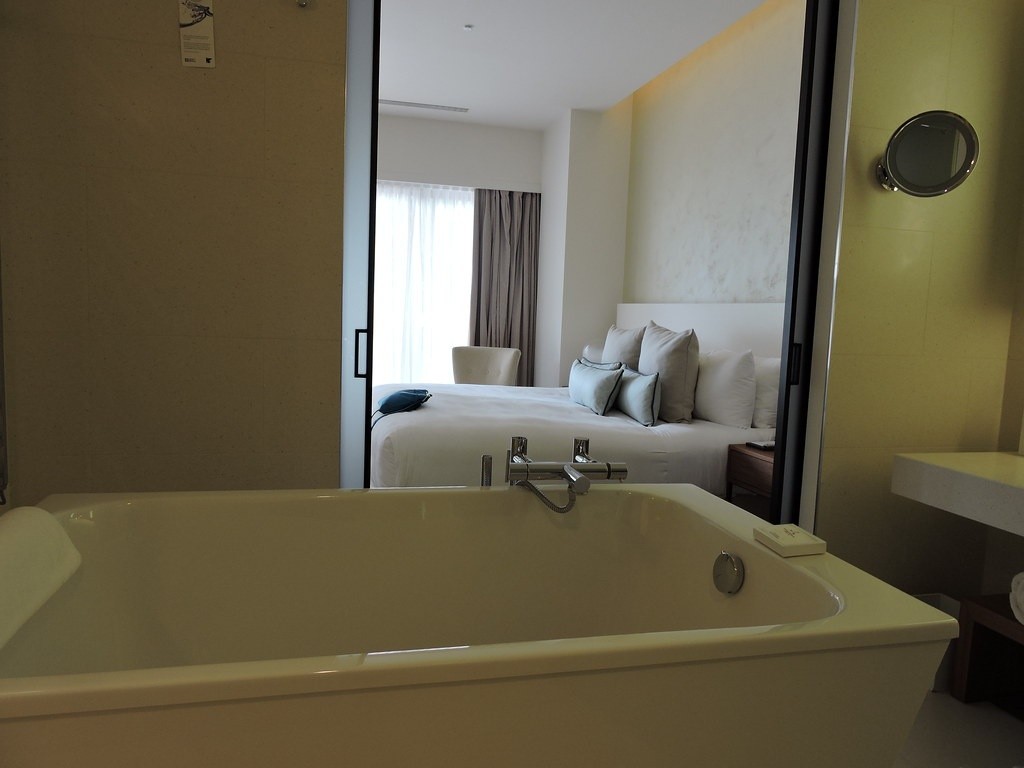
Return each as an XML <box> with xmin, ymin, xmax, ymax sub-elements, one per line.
<box><xmin>372</xmin><ymin>303</ymin><xmax>786</xmax><ymax>500</ymax></box>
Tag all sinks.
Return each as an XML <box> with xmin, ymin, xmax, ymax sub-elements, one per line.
<box><xmin>889</xmin><ymin>452</ymin><xmax>1022</xmax><ymax>535</ymax></box>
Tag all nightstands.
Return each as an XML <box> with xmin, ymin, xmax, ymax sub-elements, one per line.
<box><xmin>725</xmin><ymin>444</ymin><xmax>775</xmax><ymax>523</ymax></box>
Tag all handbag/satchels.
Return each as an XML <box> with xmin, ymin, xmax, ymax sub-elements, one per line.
<box><xmin>371</xmin><ymin>389</ymin><xmax>432</xmax><ymax>429</ymax></box>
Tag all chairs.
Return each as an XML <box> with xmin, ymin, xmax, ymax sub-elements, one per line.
<box><xmin>452</xmin><ymin>346</ymin><xmax>522</xmax><ymax>386</ymax></box>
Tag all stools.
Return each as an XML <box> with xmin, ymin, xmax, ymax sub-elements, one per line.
<box><xmin>951</xmin><ymin>594</ymin><xmax>1024</xmax><ymax>705</ymax></box>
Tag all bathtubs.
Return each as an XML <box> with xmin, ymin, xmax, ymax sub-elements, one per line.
<box><xmin>4</xmin><ymin>482</ymin><xmax>954</xmax><ymax>763</ymax></box>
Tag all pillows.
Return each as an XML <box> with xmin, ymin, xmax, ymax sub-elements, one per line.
<box><xmin>583</xmin><ymin>345</ymin><xmax>603</xmax><ymax>364</ymax></box>
<box><xmin>568</xmin><ymin>359</ymin><xmax>624</xmax><ymax>417</ymax></box>
<box><xmin>601</xmin><ymin>321</ymin><xmax>647</xmax><ymax>371</ymax></box>
<box><xmin>692</xmin><ymin>349</ymin><xmax>757</xmax><ymax>429</ymax></box>
<box><xmin>581</xmin><ymin>357</ymin><xmax>621</xmax><ymax>371</ymax></box>
<box><xmin>637</xmin><ymin>320</ymin><xmax>700</xmax><ymax>424</ymax></box>
<box><xmin>615</xmin><ymin>362</ymin><xmax>662</xmax><ymax>427</ymax></box>
<box><xmin>752</xmin><ymin>357</ymin><xmax>782</xmax><ymax>429</ymax></box>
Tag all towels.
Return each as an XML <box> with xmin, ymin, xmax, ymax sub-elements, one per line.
<box><xmin>2</xmin><ymin>506</ymin><xmax>84</xmax><ymax>660</ymax></box>
<box><xmin>1010</xmin><ymin>570</ymin><xmax>1024</xmax><ymax>627</ymax></box>
